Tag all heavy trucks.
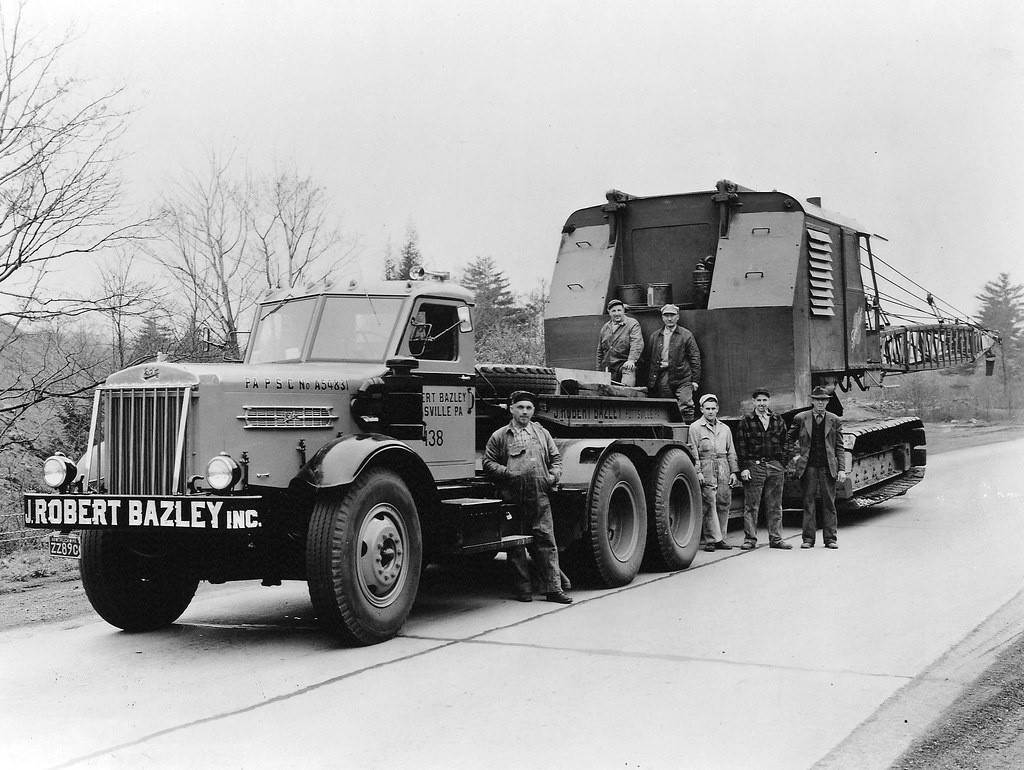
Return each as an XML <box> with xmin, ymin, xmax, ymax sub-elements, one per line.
<box><xmin>20</xmin><ymin>264</ymin><xmax>706</xmax><ymax>648</ymax></box>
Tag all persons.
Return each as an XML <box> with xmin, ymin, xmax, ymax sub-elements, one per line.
<box><xmin>788</xmin><ymin>387</ymin><xmax>846</xmax><ymax>549</ymax></box>
<box><xmin>596</xmin><ymin>300</ymin><xmax>644</xmax><ymax>386</ymax></box>
<box><xmin>640</xmin><ymin>304</ymin><xmax>701</xmax><ymax>426</ymax></box>
<box><xmin>688</xmin><ymin>394</ymin><xmax>739</xmax><ymax>551</ymax></box>
<box><xmin>736</xmin><ymin>388</ymin><xmax>793</xmax><ymax>550</ymax></box>
<box><xmin>482</xmin><ymin>391</ymin><xmax>573</xmax><ymax>604</ymax></box>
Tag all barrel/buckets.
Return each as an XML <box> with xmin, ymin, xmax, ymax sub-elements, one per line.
<box><xmin>647</xmin><ymin>282</ymin><xmax>673</xmax><ymax>307</ymax></box>
<box><xmin>617</xmin><ymin>283</ymin><xmax>642</xmax><ymax>305</ymax></box>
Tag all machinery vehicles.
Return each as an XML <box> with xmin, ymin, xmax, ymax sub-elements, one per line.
<box><xmin>542</xmin><ymin>179</ymin><xmax>1004</xmax><ymax>517</ymax></box>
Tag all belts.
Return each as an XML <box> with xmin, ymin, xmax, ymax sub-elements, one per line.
<box><xmin>753</xmin><ymin>456</ymin><xmax>776</xmax><ymax>463</ymax></box>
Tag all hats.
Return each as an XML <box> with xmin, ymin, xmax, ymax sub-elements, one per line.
<box><xmin>807</xmin><ymin>386</ymin><xmax>832</xmax><ymax>398</ymax></box>
<box><xmin>699</xmin><ymin>393</ymin><xmax>718</xmax><ymax>406</ymax></box>
<box><xmin>510</xmin><ymin>390</ymin><xmax>536</xmax><ymax>406</ymax></box>
<box><xmin>661</xmin><ymin>304</ymin><xmax>679</xmax><ymax>315</ymax></box>
<box><xmin>608</xmin><ymin>299</ymin><xmax>623</xmax><ymax>310</ymax></box>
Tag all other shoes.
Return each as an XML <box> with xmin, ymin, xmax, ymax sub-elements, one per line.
<box><xmin>741</xmin><ymin>542</ymin><xmax>754</xmax><ymax>549</ymax></box>
<box><xmin>547</xmin><ymin>591</ymin><xmax>573</xmax><ymax>603</ymax></box>
<box><xmin>825</xmin><ymin>542</ymin><xmax>837</xmax><ymax>549</ymax></box>
<box><xmin>715</xmin><ymin>540</ymin><xmax>732</xmax><ymax>549</ymax></box>
<box><xmin>801</xmin><ymin>542</ymin><xmax>814</xmax><ymax>548</ymax></box>
<box><xmin>776</xmin><ymin>541</ymin><xmax>792</xmax><ymax>549</ymax></box>
<box><xmin>517</xmin><ymin>593</ymin><xmax>532</xmax><ymax>602</ymax></box>
<box><xmin>705</xmin><ymin>543</ymin><xmax>715</xmax><ymax>551</ymax></box>
